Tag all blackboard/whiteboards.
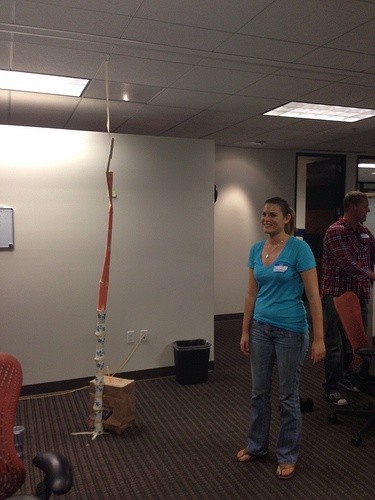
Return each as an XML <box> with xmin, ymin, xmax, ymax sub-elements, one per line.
<box><xmin>0</xmin><ymin>209</ymin><xmax>13</xmax><ymax>247</ymax></box>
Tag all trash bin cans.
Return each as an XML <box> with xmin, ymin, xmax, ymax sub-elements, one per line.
<box><xmin>172</xmin><ymin>339</ymin><xmax>212</xmax><ymax>385</ymax></box>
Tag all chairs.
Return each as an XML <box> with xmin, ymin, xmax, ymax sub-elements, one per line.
<box><xmin>326</xmin><ymin>290</ymin><xmax>375</xmax><ymax>447</ymax></box>
<box><xmin>0</xmin><ymin>351</ymin><xmax>74</xmax><ymax>500</ymax></box>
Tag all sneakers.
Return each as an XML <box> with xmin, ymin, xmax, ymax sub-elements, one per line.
<box><xmin>338</xmin><ymin>378</ymin><xmax>360</xmax><ymax>392</ymax></box>
<box><xmin>323</xmin><ymin>388</ymin><xmax>348</xmax><ymax>405</ymax></box>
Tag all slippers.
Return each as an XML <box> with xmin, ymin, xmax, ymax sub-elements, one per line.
<box><xmin>277</xmin><ymin>463</ymin><xmax>295</xmax><ymax>479</ymax></box>
<box><xmin>237</xmin><ymin>447</ymin><xmax>258</xmax><ymax>462</ymax></box>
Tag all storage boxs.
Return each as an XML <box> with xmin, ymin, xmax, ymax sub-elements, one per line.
<box><xmin>88</xmin><ymin>376</ymin><xmax>135</xmax><ymax>432</ymax></box>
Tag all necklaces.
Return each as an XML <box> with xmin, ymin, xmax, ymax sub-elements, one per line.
<box><xmin>265</xmin><ymin>234</ymin><xmax>290</xmax><ymax>259</ymax></box>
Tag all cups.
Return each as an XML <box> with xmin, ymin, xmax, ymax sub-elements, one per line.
<box><xmin>12</xmin><ymin>425</ymin><xmax>24</xmax><ymax>458</ymax></box>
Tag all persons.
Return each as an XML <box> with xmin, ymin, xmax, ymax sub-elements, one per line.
<box><xmin>320</xmin><ymin>191</ymin><xmax>375</xmax><ymax>406</ymax></box>
<box><xmin>237</xmin><ymin>197</ymin><xmax>326</xmax><ymax>481</ymax></box>
